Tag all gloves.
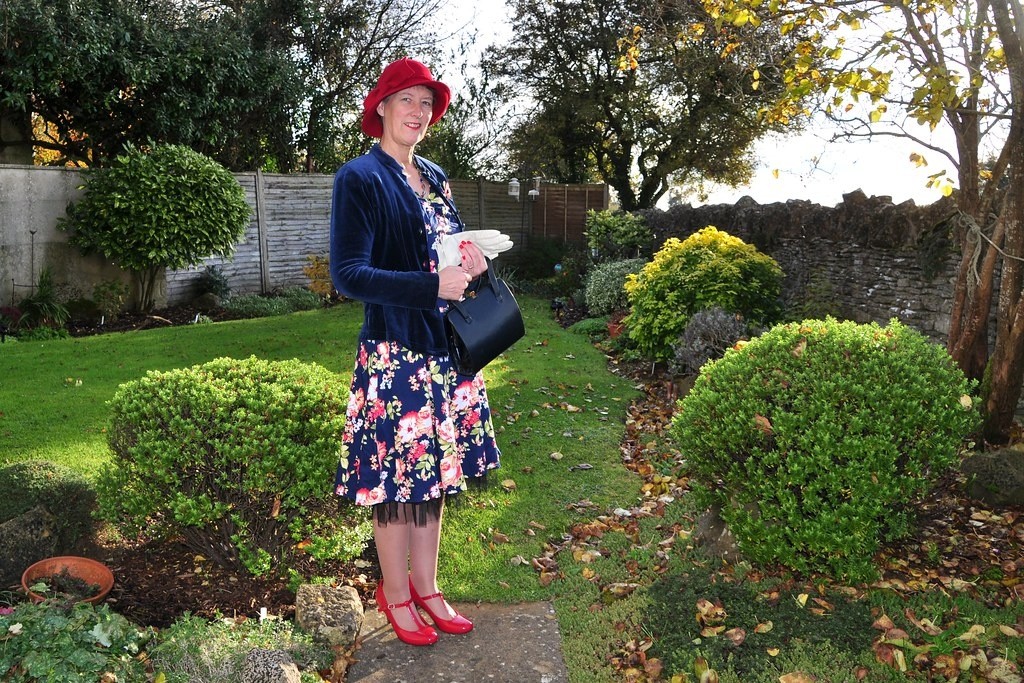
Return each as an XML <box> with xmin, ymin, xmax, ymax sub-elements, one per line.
<box><xmin>432</xmin><ymin>228</ymin><xmax>514</xmax><ymax>273</ymax></box>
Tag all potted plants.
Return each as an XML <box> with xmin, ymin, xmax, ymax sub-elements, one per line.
<box><xmin>22</xmin><ymin>556</ymin><xmax>114</xmax><ymax>609</ymax></box>
<box><xmin>585</xmin><ymin>258</ymin><xmax>650</xmax><ymax>339</ymax></box>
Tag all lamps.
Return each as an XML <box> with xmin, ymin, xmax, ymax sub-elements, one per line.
<box><xmin>508</xmin><ymin>178</ymin><xmax>520</xmax><ymax>202</ymax></box>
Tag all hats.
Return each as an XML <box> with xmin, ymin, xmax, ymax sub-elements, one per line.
<box><xmin>360</xmin><ymin>55</ymin><xmax>451</xmax><ymax>138</ymax></box>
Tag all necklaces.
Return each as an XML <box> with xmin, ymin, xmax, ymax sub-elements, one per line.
<box><xmin>411</xmin><ymin>161</ymin><xmax>426</xmax><ymax>199</ymax></box>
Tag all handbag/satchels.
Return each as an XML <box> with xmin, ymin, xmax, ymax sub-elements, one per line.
<box><xmin>442</xmin><ymin>255</ymin><xmax>526</xmax><ymax>377</ymax></box>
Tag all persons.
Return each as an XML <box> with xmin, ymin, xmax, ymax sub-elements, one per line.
<box><xmin>328</xmin><ymin>57</ymin><xmax>526</xmax><ymax>648</ymax></box>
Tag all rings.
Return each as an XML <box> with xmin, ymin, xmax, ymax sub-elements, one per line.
<box><xmin>469</xmin><ymin>265</ymin><xmax>474</xmax><ymax>269</ymax></box>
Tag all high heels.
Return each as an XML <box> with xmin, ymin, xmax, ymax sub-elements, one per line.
<box><xmin>374</xmin><ymin>576</ymin><xmax>439</xmax><ymax>646</ymax></box>
<box><xmin>406</xmin><ymin>574</ymin><xmax>474</xmax><ymax>634</ymax></box>
